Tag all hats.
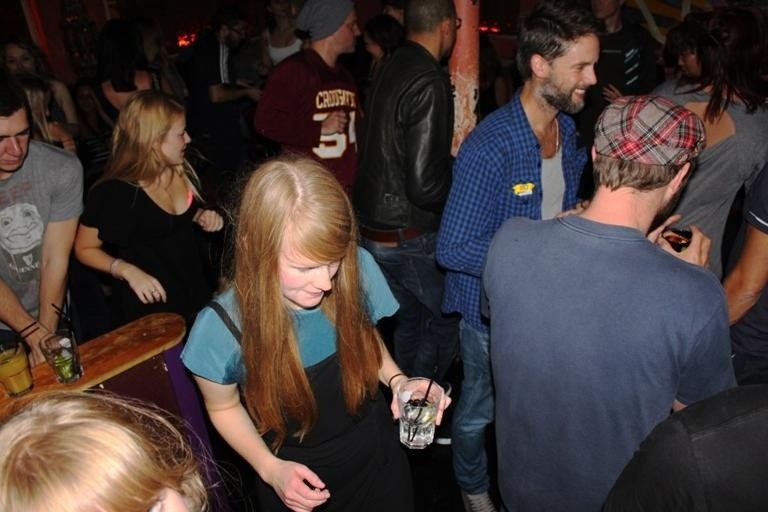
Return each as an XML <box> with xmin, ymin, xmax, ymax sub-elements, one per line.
<box><xmin>593</xmin><ymin>95</ymin><xmax>707</xmax><ymax>168</ymax></box>
<box><xmin>295</xmin><ymin>1</ymin><xmax>353</xmax><ymax>41</ymax></box>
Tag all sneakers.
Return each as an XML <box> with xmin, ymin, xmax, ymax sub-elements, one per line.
<box><xmin>461</xmin><ymin>490</ymin><xmax>496</xmax><ymax>511</ymax></box>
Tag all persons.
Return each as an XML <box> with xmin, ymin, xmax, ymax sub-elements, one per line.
<box><xmin>0</xmin><ymin>0</ymin><xmax>768</xmax><ymax>511</ymax></box>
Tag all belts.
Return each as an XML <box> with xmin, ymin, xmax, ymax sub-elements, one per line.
<box><xmin>357</xmin><ymin>223</ymin><xmax>424</xmax><ymax>243</ymax></box>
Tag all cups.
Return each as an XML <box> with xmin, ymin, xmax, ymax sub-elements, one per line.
<box><xmin>0</xmin><ymin>330</ymin><xmax>82</xmax><ymax>398</ymax></box>
<box><xmin>396</xmin><ymin>376</ymin><xmax>443</xmax><ymax>450</ymax></box>
